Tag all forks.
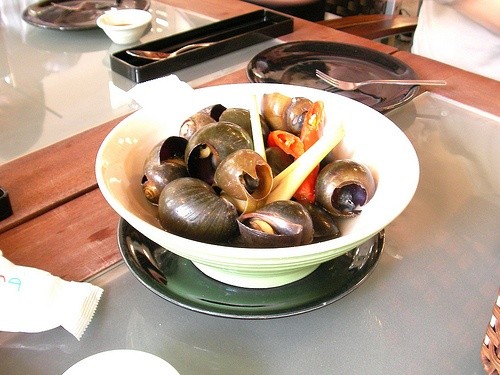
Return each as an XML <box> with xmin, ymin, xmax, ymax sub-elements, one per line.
<box><xmin>50</xmin><ymin>1</ymin><xmax>118</xmax><ymax>12</ymax></box>
<box><xmin>315</xmin><ymin>68</ymin><xmax>447</xmax><ymax>92</ymax></box>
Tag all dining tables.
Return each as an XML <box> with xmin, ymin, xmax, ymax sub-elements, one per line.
<box><xmin>0</xmin><ymin>0</ymin><xmax>500</xmax><ymax>375</ymax></box>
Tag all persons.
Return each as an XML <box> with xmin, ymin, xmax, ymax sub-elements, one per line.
<box><xmin>410</xmin><ymin>0</ymin><xmax>500</xmax><ymax>82</ymax></box>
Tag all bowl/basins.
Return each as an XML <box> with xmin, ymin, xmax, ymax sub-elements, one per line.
<box><xmin>94</xmin><ymin>83</ymin><xmax>419</xmax><ymax>290</ymax></box>
<box><xmin>95</xmin><ymin>9</ymin><xmax>152</xmax><ymax>44</ymax></box>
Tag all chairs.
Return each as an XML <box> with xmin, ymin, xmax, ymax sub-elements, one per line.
<box><xmin>338</xmin><ymin>0</ymin><xmax>500</xmax><ymax>82</ymax></box>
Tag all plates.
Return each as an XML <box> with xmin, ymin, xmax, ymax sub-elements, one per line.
<box><xmin>116</xmin><ymin>216</ymin><xmax>385</xmax><ymax>320</ymax></box>
<box><xmin>20</xmin><ymin>0</ymin><xmax>150</xmax><ymax>30</ymax></box>
<box><xmin>245</xmin><ymin>39</ymin><xmax>419</xmax><ymax>114</ymax></box>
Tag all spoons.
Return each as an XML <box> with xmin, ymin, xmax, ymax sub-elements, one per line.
<box><xmin>125</xmin><ymin>30</ymin><xmax>270</xmax><ymax>61</ymax></box>
